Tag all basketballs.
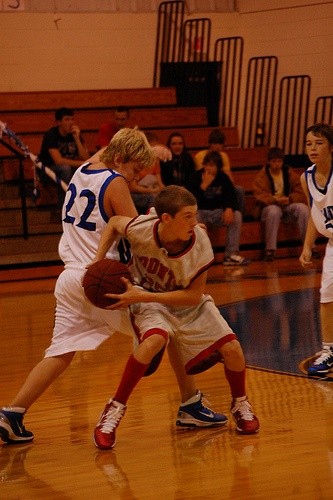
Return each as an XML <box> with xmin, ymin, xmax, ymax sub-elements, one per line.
<box><xmin>83</xmin><ymin>259</ymin><xmax>134</xmax><ymax>310</ymax></box>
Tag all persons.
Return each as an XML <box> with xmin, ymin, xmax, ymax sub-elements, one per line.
<box><xmin>299</xmin><ymin>123</ymin><xmax>333</xmax><ymax>373</ymax></box>
<box><xmin>81</xmin><ymin>185</ymin><xmax>261</xmax><ymax>449</ymax></box>
<box><xmin>161</xmin><ymin>131</ymin><xmax>197</xmax><ymax>192</ymax></box>
<box><xmin>97</xmin><ymin>105</ymin><xmax>131</xmax><ymax>149</ymax></box>
<box><xmin>37</xmin><ymin>106</ymin><xmax>87</xmax><ymax>208</ymax></box>
<box><xmin>193</xmin><ymin>130</ymin><xmax>233</xmax><ymax>183</ymax></box>
<box><xmin>183</xmin><ymin>150</ymin><xmax>251</xmax><ymax>266</ymax></box>
<box><xmin>253</xmin><ymin>148</ymin><xmax>321</xmax><ymax>263</ymax></box>
<box><xmin>0</xmin><ymin>129</ymin><xmax>229</xmax><ymax>448</ymax></box>
<box><xmin>127</xmin><ymin>133</ymin><xmax>166</xmax><ymax>209</ymax></box>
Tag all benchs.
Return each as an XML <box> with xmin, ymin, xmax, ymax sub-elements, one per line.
<box><xmin>0</xmin><ymin>85</ymin><xmax>331</xmax><ymax>282</ymax></box>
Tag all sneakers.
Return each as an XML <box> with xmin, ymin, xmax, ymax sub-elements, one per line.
<box><xmin>0</xmin><ymin>407</ymin><xmax>34</xmax><ymax>443</ymax></box>
<box><xmin>223</xmin><ymin>254</ymin><xmax>250</xmax><ymax>265</ymax></box>
<box><xmin>307</xmin><ymin>342</ymin><xmax>333</xmax><ymax>380</ymax></box>
<box><xmin>229</xmin><ymin>392</ymin><xmax>260</xmax><ymax>433</ymax></box>
<box><xmin>176</xmin><ymin>390</ymin><xmax>229</xmax><ymax>430</ymax></box>
<box><xmin>93</xmin><ymin>397</ymin><xmax>128</xmax><ymax>449</ymax></box>
